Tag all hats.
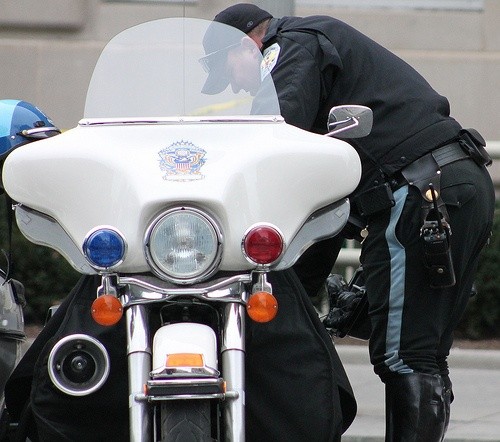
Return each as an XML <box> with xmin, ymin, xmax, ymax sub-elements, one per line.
<box><xmin>202</xmin><ymin>2</ymin><xmax>275</xmax><ymax>96</ymax></box>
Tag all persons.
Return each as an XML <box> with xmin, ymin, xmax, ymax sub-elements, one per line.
<box><xmin>202</xmin><ymin>4</ymin><xmax>497</xmax><ymax>441</ymax></box>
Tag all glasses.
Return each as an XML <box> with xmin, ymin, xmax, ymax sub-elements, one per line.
<box><xmin>199</xmin><ymin>42</ymin><xmax>242</xmax><ymax>74</ymax></box>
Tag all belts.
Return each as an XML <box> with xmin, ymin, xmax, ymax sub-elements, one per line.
<box><xmin>381</xmin><ymin>144</ymin><xmax>470</xmax><ymax>194</ymax></box>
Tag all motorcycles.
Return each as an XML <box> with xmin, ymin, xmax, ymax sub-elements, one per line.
<box><xmin>0</xmin><ymin>16</ymin><xmax>375</xmax><ymax>441</ymax></box>
